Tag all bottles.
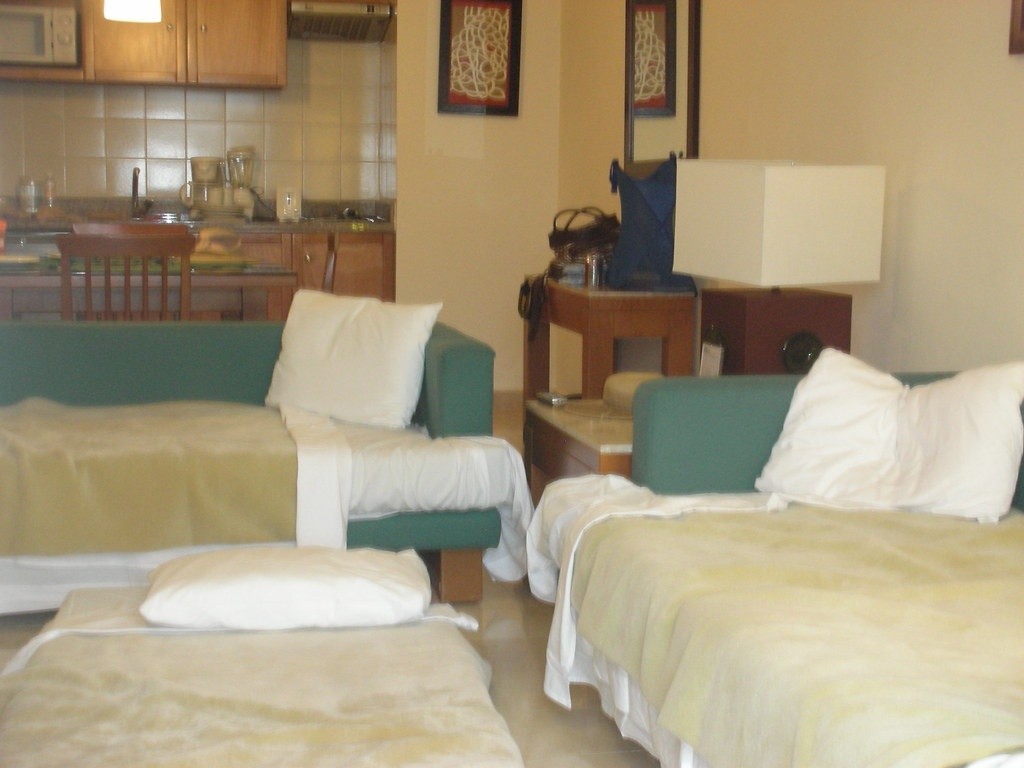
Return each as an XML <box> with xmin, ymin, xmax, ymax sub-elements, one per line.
<box><xmin>44</xmin><ymin>172</ymin><xmax>57</xmax><ymax>208</ymax></box>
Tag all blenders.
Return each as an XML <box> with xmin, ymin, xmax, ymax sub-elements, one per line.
<box><xmin>180</xmin><ymin>145</ymin><xmax>257</xmax><ymax>223</ymax></box>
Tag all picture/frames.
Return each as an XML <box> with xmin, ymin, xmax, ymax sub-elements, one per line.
<box><xmin>632</xmin><ymin>1</ymin><xmax>676</xmax><ymax>116</ymax></box>
<box><xmin>438</xmin><ymin>0</ymin><xmax>522</xmax><ymax>117</ymax></box>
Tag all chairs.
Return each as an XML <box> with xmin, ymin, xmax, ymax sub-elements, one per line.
<box><xmin>55</xmin><ymin>223</ymin><xmax>195</xmax><ymax>320</ymax></box>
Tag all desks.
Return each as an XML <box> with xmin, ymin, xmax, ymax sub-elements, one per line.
<box><xmin>0</xmin><ymin>317</ymin><xmax>534</xmax><ymax>615</ymax></box>
<box><xmin>523</xmin><ymin>278</ymin><xmax>694</xmax><ymax>401</ymax></box>
<box><xmin>0</xmin><ymin>273</ymin><xmax>299</xmax><ymax>321</ymax></box>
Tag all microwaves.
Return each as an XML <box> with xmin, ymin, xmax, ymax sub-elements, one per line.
<box><xmin>0</xmin><ymin>5</ymin><xmax>77</xmax><ymax>68</ymax></box>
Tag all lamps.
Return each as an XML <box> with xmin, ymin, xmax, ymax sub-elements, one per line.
<box><xmin>671</xmin><ymin>157</ymin><xmax>886</xmax><ymax>375</ymax></box>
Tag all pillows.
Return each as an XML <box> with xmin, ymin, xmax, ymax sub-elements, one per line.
<box><xmin>266</xmin><ymin>289</ymin><xmax>443</xmax><ymax>430</ymax></box>
<box><xmin>140</xmin><ymin>546</ymin><xmax>430</xmax><ymax>628</ymax></box>
<box><xmin>754</xmin><ymin>346</ymin><xmax>1024</xmax><ymax>522</ymax></box>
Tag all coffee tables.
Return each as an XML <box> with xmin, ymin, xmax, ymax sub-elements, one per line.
<box><xmin>0</xmin><ymin>584</ymin><xmax>524</xmax><ymax>767</ymax></box>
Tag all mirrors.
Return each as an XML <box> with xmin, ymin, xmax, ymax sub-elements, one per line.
<box><xmin>624</xmin><ymin>0</ymin><xmax>701</xmax><ymax>179</ymax></box>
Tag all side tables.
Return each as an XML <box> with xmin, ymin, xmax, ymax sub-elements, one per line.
<box><xmin>523</xmin><ymin>396</ymin><xmax>634</xmax><ymax>582</ymax></box>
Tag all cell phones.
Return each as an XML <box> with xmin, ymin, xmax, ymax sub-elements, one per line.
<box><xmin>536</xmin><ymin>390</ymin><xmax>569</xmax><ymax>406</ymax></box>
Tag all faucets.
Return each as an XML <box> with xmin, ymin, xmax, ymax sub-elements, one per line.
<box><xmin>131</xmin><ymin>167</ymin><xmax>153</xmax><ymax>220</ymax></box>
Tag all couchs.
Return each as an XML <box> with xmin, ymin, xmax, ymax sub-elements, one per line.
<box><xmin>526</xmin><ymin>372</ymin><xmax>1024</xmax><ymax>768</ymax></box>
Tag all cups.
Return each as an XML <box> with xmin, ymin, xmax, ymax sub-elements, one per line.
<box><xmin>586</xmin><ymin>253</ymin><xmax>602</xmax><ymax>286</ymax></box>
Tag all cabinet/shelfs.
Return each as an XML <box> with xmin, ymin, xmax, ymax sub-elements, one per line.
<box><xmin>80</xmin><ymin>0</ymin><xmax>289</xmax><ymax>88</ymax></box>
<box><xmin>196</xmin><ymin>234</ymin><xmax>395</xmax><ymax>320</ymax></box>
